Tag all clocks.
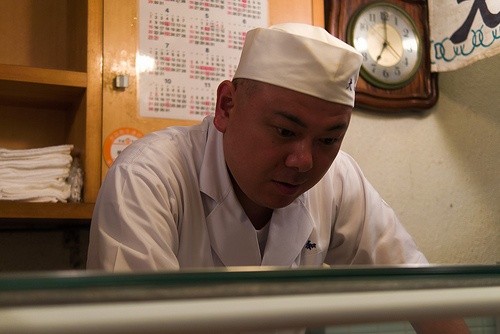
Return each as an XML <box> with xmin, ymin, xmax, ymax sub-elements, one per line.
<box><xmin>322</xmin><ymin>1</ymin><xmax>437</xmax><ymax>113</ymax></box>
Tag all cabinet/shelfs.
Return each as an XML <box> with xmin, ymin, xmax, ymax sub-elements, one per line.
<box><xmin>0</xmin><ymin>0</ymin><xmax>326</xmax><ymax>227</ymax></box>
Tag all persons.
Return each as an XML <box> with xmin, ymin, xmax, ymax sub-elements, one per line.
<box><xmin>87</xmin><ymin>23</ymin><xmax>472</xmax><ymax>334</ymax></box>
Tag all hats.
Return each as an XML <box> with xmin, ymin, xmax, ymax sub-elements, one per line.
<box><xmin>233</xmin><ymin>22</ymin><xmax>364</xmax><ymax>109</ymax></box>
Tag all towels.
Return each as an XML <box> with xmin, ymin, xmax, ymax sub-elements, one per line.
<box><xmin>1</xmin><ymin>144</ymin><xmax>75</xmax><ymax>204</ymax></box>
